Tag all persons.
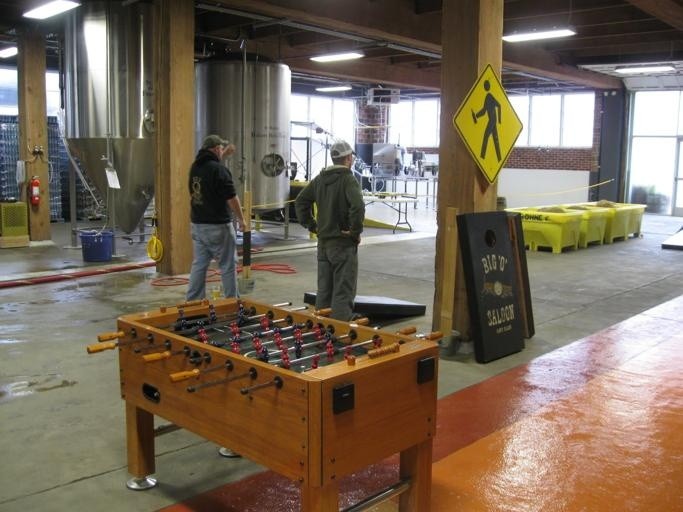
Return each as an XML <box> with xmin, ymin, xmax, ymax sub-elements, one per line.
<box><xmin>293</xmin><ymin>140</ymin><xmax>366</xmax><ymax>322</ymax></box>
<box><xmin>183</xmin><ymin>133</ymin><xmax>246</xmax><ymax>304</ymax></box>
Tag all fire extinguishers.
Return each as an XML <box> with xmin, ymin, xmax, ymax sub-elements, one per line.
<box><xmin>27</xmin><ymin>175</ymin><xmax>40</xmax><ymax>205</ymax></box>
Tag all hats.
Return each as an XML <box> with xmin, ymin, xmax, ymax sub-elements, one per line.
<box><xmin>201</xmin><ymin>134</ymin><xmax>229</xmax><ymax>148</ymax></box>
<box><xmin>330</xmin><ymin>139</ymin><xmax>357</xmax><ymax>158</ymax></box>
